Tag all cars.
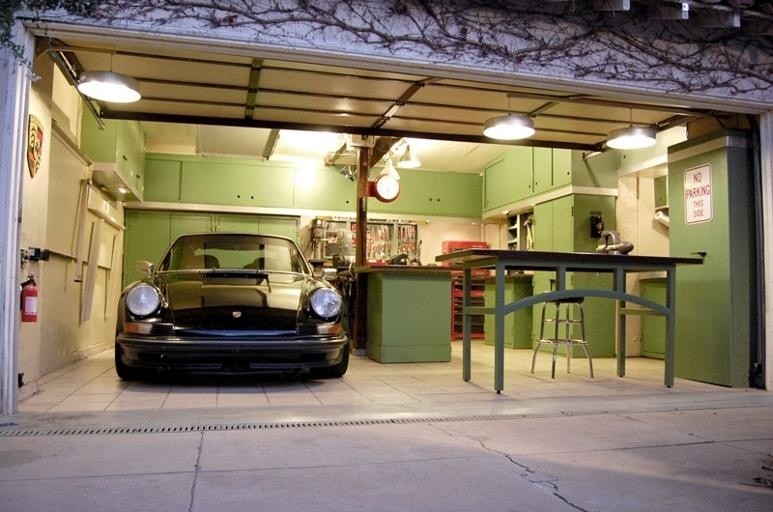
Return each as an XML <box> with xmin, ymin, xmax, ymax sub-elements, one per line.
<box><xmin>115</xmin><ymin>229</ymin><xmax>349</xmax><ymax>387</ymax></box>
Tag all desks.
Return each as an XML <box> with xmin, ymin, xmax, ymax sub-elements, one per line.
<box><xmin>355</xmin><ymin>264</ymin><xmax>454</xmax><ymax>364</ymax></box>
<box><xmin>436</xmin><ymin>247</ymin><xmax>702</xmax><ymax>395</ymax></box>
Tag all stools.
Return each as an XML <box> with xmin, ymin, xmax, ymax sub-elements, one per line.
<box><xmin>533</xmin><ymin>289</ymin><xmax>595</xmax><ymax>383</ymax></box>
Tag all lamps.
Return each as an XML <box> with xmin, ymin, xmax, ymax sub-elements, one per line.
<box><xmin>379</xmin><ymin>157</ymin><xmax>400</xmax><ymax>182</ymax></box>
<box><xmin>481</xmin><ymin>96</ymin><xmax>538</xmax><ymax>144</ymax></box>
<box><xmin>606</xmin><ymin>106</ymin><xmax>656</xmax><ymax>152</ymax></box>
<box><xmin>398</xmin><ymin>141</ymin><xmax>420</xmax><ymax>169</ymax></box>
<box><xmin>72</xmin><ymin>50</ymin><xmax>143</xmax><ymax>113</ymax></box>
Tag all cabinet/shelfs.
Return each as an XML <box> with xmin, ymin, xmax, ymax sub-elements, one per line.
<box><xmin>369</xmin><ymin>169</ymin><xmax>482</xmax><ymax>216</ymax></box>
<box><xmin>215</xmin><ymin>212</ymin><xmax>300</xmax><ymax>271</ymax></box>
<box><xmin>140</xmin><ymin>150</ymin><xmax>292</xmax><ymax>210</ymax></box>
<box><xmin>500</xmin><ymin>213</ymin><xmax>533</xmax><ymax>277</ymax></box>
<box><xmin>530</xmin><ymin>195</ymin><xmax>614</xmax><ymax>359</ymax></box>
<box><xmin>308</xmin><ymin>215</ymin><xmax>422</xmax><ymax>269</ymax></box>
<box><xmin>294</xmin><ymin>163</ymin><xmax>361</xmax><ymax>214</ymax></box>
<box><xmin>122</xmin><ymin>213</ymin><xmax>215</xmax><ymax>299</ymax></box>
<box><xmin>482</xmin><ymin>144</ymin><xmax>534</xmax><ymax>212</ymax></box>
<box><xmin>535</xmin><ymin>146</ymin><xmax>620</xmax><ymax>194</ymax></box>
<box><xmin>80</xmin><ymin>96</ymin><xmax>146</xmax><ymax>202</ymax></box>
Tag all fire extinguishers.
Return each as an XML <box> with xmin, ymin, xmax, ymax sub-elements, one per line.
<box><xmin>20</xmin><ymin>274</ymin><xmax>37</xmax><ymax>322</ymax></box>
